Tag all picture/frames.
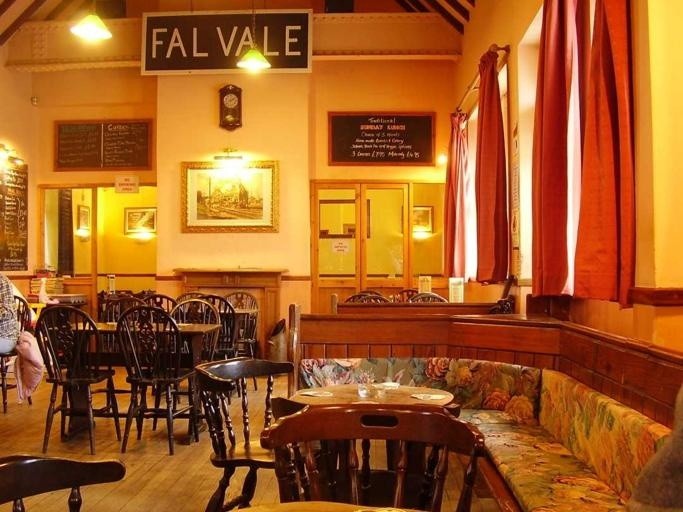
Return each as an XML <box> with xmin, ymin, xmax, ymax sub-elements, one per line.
<box><xmin>320</xmin><ymin>200</ymin><xmax>371</xmax><ymax>242</ymax></box>
<box><xmin>76</xmin><ymin>205</ymin><xmax>90</xmax><ymax>232</ymax></box>
<box><xmin>122</xmin><ymin>206</ymin><xmax>157</xmax><ymax>236</ymax></box>
<box><xmin>400</xmin><ymin>205</ymin><xmax>436</xmax><ymax>235</ymax></box>
<box><xmin>178</xmin><ymin>160</ymin><xmax>281</xmax><ymax>234</ymax></box>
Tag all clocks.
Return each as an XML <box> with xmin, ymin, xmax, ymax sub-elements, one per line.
<box><xmin>218</xmin><ymin>82</ymin><xmax>242</xmax><ymax>132</ymax></box>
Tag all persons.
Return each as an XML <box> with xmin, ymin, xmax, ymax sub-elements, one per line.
<box><xmin>0</xmin><ymin>274</ymin><xmax>17</xmax><ymax>354</ymax></box>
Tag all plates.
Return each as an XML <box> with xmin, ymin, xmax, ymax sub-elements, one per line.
<box><xmin>381</xmin><ymin>381</ymin><xmax>400</xmax><ymax>389</ymax></box>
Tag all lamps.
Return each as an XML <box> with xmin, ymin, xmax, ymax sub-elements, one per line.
<box><xmin>237</xmin><ymin>2</ymin><xmax>272</xmax><ymax>77</ymax></box>
<box><xmin>68</xmin><ymin>0</ymin><xmax>111</xmax><ymax>46</ymax></box>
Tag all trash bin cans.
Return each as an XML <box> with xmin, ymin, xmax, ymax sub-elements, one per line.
<box><xmin>268</xmin><ymin>319</ymin><xmax>289</xmax><ymax>363</ymax></box>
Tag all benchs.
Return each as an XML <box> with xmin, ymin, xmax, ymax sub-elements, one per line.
<box><xmin>284</xmin><ymin>304</ymin><xmax>682</xmax><ymax>511</ymax></box>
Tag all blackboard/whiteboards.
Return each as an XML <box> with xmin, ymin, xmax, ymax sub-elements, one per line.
<box><xmin>0</xmin><ymin>156</ymin><xmax>28</xmax><ymax>272</ymax></box>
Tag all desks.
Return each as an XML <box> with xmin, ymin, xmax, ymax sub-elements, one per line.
<box><xmin>288</xmin><ymin>382</ymin><xmax>454</xmax><ymax>506</ymax></box>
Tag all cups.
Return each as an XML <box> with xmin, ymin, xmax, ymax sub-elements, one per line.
<box><xmin>355</xmin><ymin>381</ymin><xmax>371</xmax><ymax>397</ymax></box>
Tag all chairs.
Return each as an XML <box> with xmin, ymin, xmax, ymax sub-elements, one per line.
<box><xmin>346</xmin><ymin>289</ymin><xmax>448</xmax><ymax>304</ymax></box>
<box><xmin>33</xmin><ymin>305</ymin><xmax>120</xmax><ymax>454</ymax></box>
<box><xmin>114</xmin><ymin>305</ymin><xmax>200</xmax><ymax>454</ymax></box>
<box><xmin>101</xmin><ymin>291</ymin><xmax>261</xmax><ymax>395</ymax></box>
<box><xmin>194</xmin><ymin>356</ymin><xmax>303</xmax><ymax>512</ymax></box>
<box><xmin>1</xmin><ymin>453</ymin><xmax>125</xmax><ymax>512</ymax></box>
<box><xmin>259</xmin><ymin>405</ymin><xmax>482</xmax><ymax>511</ymax></box>
